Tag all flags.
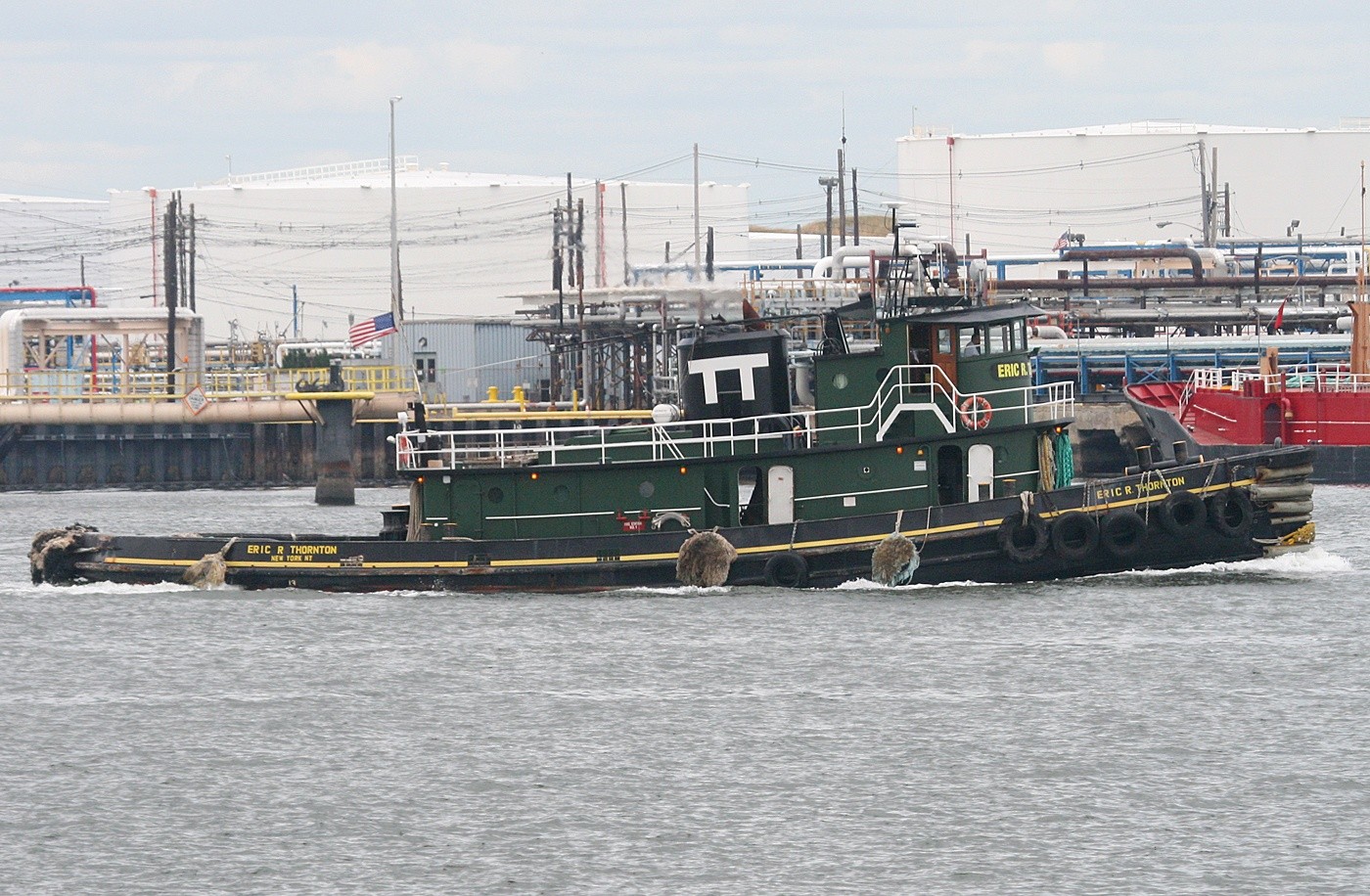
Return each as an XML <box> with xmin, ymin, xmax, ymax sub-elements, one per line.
<box><xmin>1274</xmin><ymin>302</ymin><xmax>1284</xmax><ymax>330</ymax></box>
<box><xmin>349</xmin><ymin>314</ymin><xmax>395</xmax><ymax>348</ymax></box>
<box><xmin>1052</xmin><ymin>231</ymin><xmax>1068</xmax><ymax>252</ymax></box>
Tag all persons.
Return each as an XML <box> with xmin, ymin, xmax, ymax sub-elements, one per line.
<box><xmin>964</xmin><ymin>333</ymin><xmax>981</xmax><ymax>356</ymax></box>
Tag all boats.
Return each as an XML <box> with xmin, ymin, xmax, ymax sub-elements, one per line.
<box><xmin>32</xmin><ymin>238</ymin><xmax>1317</xmax><ymax>594</ymax></box>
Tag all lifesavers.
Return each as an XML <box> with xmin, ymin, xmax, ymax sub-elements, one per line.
<box><xmin>765</xmin><ymin>549</ymin><xmax>808</xmax><ymax>588</ymax></box>
<box><xmin>960</xmin><ymin>397</ymin><xmax>991</xmax><ymax>430</ymax></box>
<box><xmin>400</xmin><ymin>438</ymin><xmax>409</xmax><ymax>465</ymax></box>
<box><xmin>995</xmin><ymin>487</ymin><xmax>1253</xmax><ymax>563</ymax></box>
